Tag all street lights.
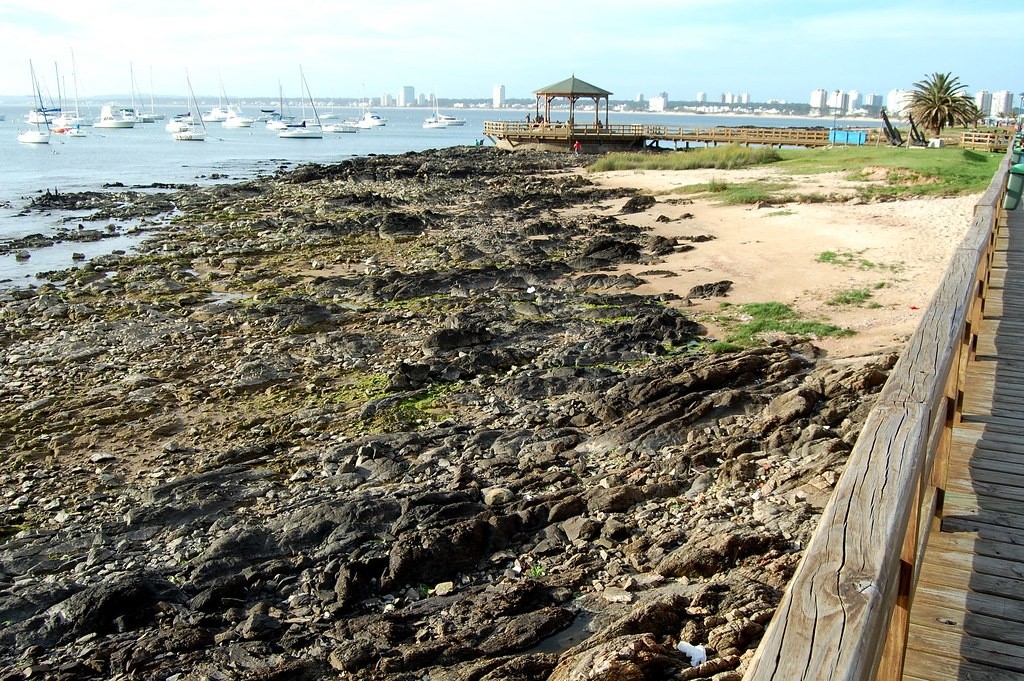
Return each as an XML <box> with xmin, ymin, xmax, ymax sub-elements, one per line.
<box><xmin>834</xmin><ymin>89</ymin><xmax>840</xmax><ymax>128</ymax></box>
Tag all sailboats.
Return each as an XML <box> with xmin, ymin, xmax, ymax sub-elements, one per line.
<box><xmin>17</xmin><ymin>49</ymin><xmax>87</xmax><ymax>143</ymax></box>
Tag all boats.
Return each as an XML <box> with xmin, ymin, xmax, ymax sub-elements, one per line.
<box><xmin>201</xmin><ymin>71</ymin><xmax>254</xmax><ymax>127</ymax></box>
<box><xmin>318</xmin><ymin>92</ymin><xmax>342</xmax><ymax>119</ymax></box>
<box><xmin>165</xmin><ymin>75</ymin><xmax>207</xmax><ymax>141</ymax></box>
<box><xmin>422</xmin><ymin>88</ymin><xmax>467</xmax><ymax>128</ymax></box>
<box><xmin>321</xmin><ymin>83</ymin><xmax>388</xmax><ymax>133</ymax></box>
<box><xmin>92</xmin><ymin>60</ymin><xmax>165</xmax><ymax>128</ymax></box>
<box><xmin>256</xmin><ymin>63</ymin><xmax>323</xmax><ymax>139</ymax></box>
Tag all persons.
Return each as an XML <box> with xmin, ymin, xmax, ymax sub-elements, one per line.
<box><xmin>526</xmin><ymin>113</ymin><xmax>530</xmax><ymax>123</ymax></box>
<box><xmin>1020</xmin><ymin>136</ymin><xmax>1024</xmax><ymax>155</ymax></box>
<box><xmin>598</xmin><ymin>120</ymin><xmax>603</xmax><ymax>128</ymax></box>
<box><xmin>574</xmin><ymin>140</ymin><xmax>581</xmax><ymax>156</ymax></box>
<box><xmin>984</xmin><ymin>130</ymin><xmax>1009</xmax><ymax>144</ymax></box>
<box><xmin>476</xmin><ymin>139</ymin><xmax>483</xmax><ymax>146</ymax></box>
<box><xmin>533</xmin><ymin>113</ymin><xmax>544</xmax><ymax>123</ymax></box>
